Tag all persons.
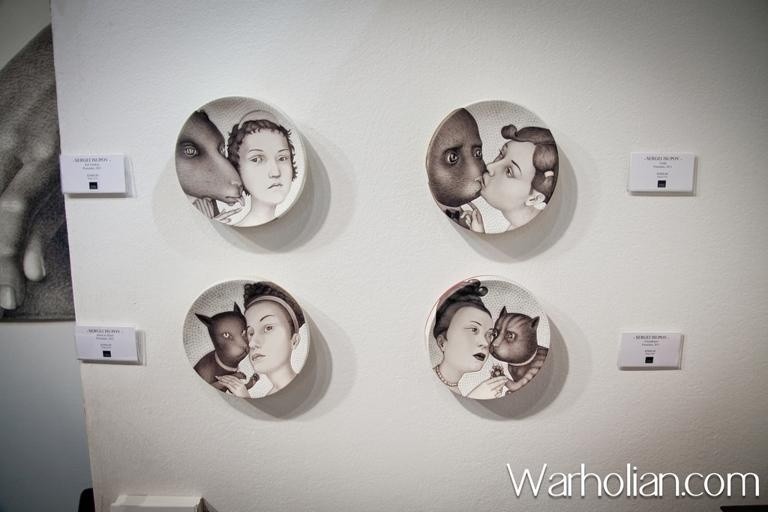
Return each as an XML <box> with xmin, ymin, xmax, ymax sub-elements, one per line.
<box><xmin>214</xmin><ymin>284</ymin><xmax>305</xmax><ymax>400</ymax></box>
<box><xmin>462</xmin><ymin>123</ymin><xmax>558</xmax><ymax>235</ymax></box>
<box><xmin>210</xmin><ymin>114</ymin><xmax>297</xmax><ymax>228</ymax></box>
<box><xmin>1</xmin><ymin>24</ymin><xmax>67</xmax><ymax>314</ymax></box>
<box><xmin>431</xmin><ymin>278</ymin><xmax>509</xmax><ymax>403</ymax></box>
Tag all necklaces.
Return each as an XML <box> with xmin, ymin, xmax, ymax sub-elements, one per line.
<box><xmin>436</xmin><ymin>364</ymin><xmax>462</xmax><ymax>391</ymax></box>
